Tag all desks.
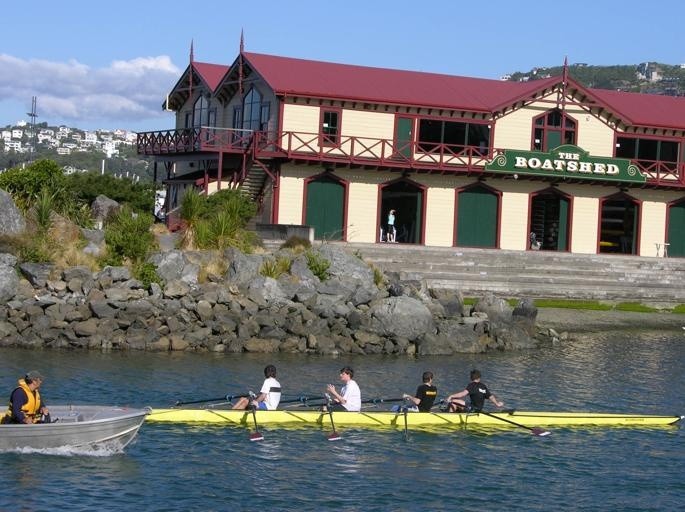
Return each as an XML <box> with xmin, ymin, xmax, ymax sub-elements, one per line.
<box><xmin>654</xmin><ymin>242</ymin><xmax>670</xmax><ymax>258</ymax></box>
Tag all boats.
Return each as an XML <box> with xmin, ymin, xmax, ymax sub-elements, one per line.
<box><xmin>145</xmin><ymin>406</ymin><xmax>684</xmax><ymax>433</ymax></box>
<box><xmin>1</xmin><ymin>403</ymin><xmax>152</xmax><ymax>458</ymax></box>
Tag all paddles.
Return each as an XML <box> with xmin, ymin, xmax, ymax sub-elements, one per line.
<box><xmin>251</xmin><ymin>397</ymin><xmax>265</xmax><ymax>443</ymax></box>
<box><xmin>327</xmin><ymin>402</ymin><xmax>341</xmax><ymax>441</ymax></box>
<box><xmin>448</xmin><ymin>402</ymin><xmax>552</xmax><ymax>437</ymax></box>
<box><xmin>403</xmin><ymin>397</ymin><xmax>411</xmax><ymax>442</ymax></box>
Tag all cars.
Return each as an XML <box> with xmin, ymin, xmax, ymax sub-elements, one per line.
<box><xmin>155</xmin><ymin>207</ymin><xmax>167</xmax><ymax>224</ymax></box>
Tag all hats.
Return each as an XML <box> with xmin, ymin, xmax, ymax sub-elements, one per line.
<box><xmin>27</xmin><ymin>370</ymin><xmax>43</xmax><ymax>381</ymax></box>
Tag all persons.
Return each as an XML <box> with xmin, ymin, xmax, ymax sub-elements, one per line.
<box><xmin>386</xmin><ymin>209</ymin><xmax>397</xmax><ymax>243</ymax></box>
<box><xmin>232</xmin><ymin>364</ymin><xmax>281</xmax><ymax>410</ymax></box>
<box><xmin>3</xmin><ymin>371</ymin><xmax>51</xmax><ymax>425</ymax></box>
<box><xmin>389</xmin><ymin>372</ymin><xmax>437</xmax><ymax>413</ymax></box>
<box><xmin>157</xmin><ymin>208</ymin><xmax>165</xmax><ymax>224</ymax></box>
<box><xmin>445</xmin><ymin>369</ymin><xmax>504</xmax><ymax>413</ymax></box>
<box><xmin>318</xmin><ymin>366</ymin><xmax>361</xmax><ymax>412</ymax></box>
